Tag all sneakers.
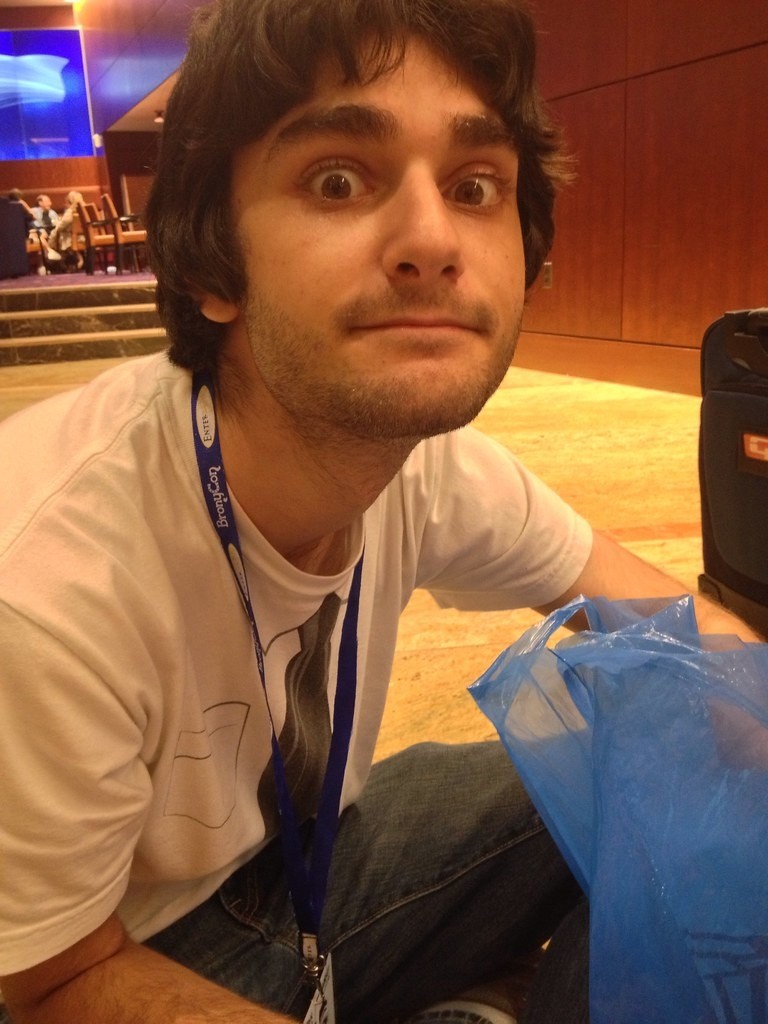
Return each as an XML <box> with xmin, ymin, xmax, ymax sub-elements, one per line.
<box><xmin>407</xmin><ymin>1000</ymin><xmax>518</xmax><ymax>1024</ymax></box>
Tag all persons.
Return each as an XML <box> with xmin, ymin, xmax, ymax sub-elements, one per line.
<box><xmin>0</xmin><ymin>188</ymin><xmax>83</xmax><ymax>278</ymax></box>
<box><xmin>2</xmin><ymin>0</ymin><xmax>765</xmax><ymax>1024</ymax></box>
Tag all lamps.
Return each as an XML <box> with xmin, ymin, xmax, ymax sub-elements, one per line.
<box><xmin>155</xmin><ymin>109</ymin><xmax>164</xmax><ymax>123</ymax></box>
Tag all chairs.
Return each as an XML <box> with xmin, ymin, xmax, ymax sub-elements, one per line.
<box><xmin>60</xmin><ymin>193</ymin><xmax>149</xmax><ymax>276</ymax></box>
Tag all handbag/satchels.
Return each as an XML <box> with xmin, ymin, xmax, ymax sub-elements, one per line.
<box><xmin>467</xmin><ymin>594</ymin><xmax>768</xmax><ymax>1024</ymax></box>
